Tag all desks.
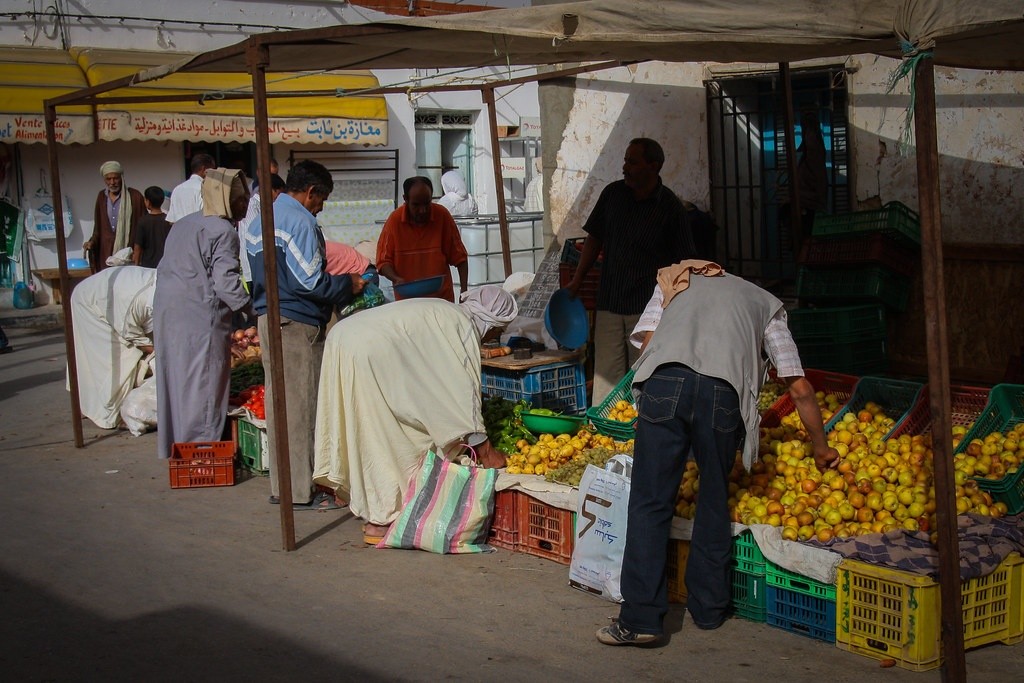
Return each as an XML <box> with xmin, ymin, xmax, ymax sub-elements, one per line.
<box><xmin>31</xmin><ymin>268</ymin><xmax>93</xmax><ymax>304</ymax></box>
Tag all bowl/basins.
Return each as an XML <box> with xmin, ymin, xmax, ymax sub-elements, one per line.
<box><xmin>545</xmin><ymin>288</ymin><xmax>589</xmax><ymax>349</ymax></box>
<box><xmin>67</xmin><ymin>258</ymin><xmax>90</xmax><ymax>270</ymax></box>
<box><xmin>520</xmin><ymin>410</ymin><xmax>586</xmax><ymax>435</ymax></box>
<box><xmin>390</xmin><ymin>275</ymin><xmax>443</xmax><ymax>297</ymax></box>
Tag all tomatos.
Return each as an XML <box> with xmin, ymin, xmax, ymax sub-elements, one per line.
<box><xmin>229</xmin><ymin>384</ymin><xmax>265</xmax><ymax>419</ymax></box>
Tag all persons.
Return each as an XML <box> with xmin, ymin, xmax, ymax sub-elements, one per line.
<box><xmin>84</xmin><ymin>161</ymin><xmax>147</xmax><ymax>274</ymax></box>
<box><xmin>325</xmin><ymin>241</ymin><xmax>379</xmax><ymax>295</ymax></box>
<box><xmin>564</xmin><ymin>137</ymin><xmax>699</xmax><ymax>410</ymax></box>
<box><xmin>249</xmin><ymin>159</ymin><xmax>278</xmax><ymax>195</ymax></box>
<box><xmin>312</xmin><ymin>284</ymin><xmax>519</xmax><ymax>544</ymax></box>
<box><xmin>376</xmin><ymin>177</ymin><xmax>469</xmax><ymax>304</ymax></box>
<box><xmin>526</xmin><ymin>157</ymin><xmax>543</xmax><ymax>211</ymax></box>
<box><xmin>596</xmin><ymin>260</ymin><xmax>840</xmax><ymax>644</ymax></box>
<box><xmin>238</xmin><ymin>173</ymin><xmax>285</xmax><ymax>282</ymax></box>
<box><xmin>247</xmin><ymin>159</ymin><xmax>366</xmax><ymax>511</ymax></box>
<box><xmin>436</xmin><ymin>171</ymin><xmax>478</xmax><ymax>215</ymax></box>
<box><xmin>132</xmin><ymin>186</ymin><xmax>171</xmax><ymax>267</ymax></box>
<box><xmin>66</xmin><ymin>266</ymin><xmax>157</xmax><ymax>429</ymax></box>
<box><xmin>152</xmin><ymin>169</ymin><xmax>252</xmax><ymax>461</ymax></box>
<box><xmin>166</xmin><ymin>154</ymin><xmax>215</xmax><ymax>224</ymax></box>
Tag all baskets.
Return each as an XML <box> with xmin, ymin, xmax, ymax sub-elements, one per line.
<box><xmin>168</xmin><ymin>440</ymin><xmax>235</xmax><ymax>489</ymax></box>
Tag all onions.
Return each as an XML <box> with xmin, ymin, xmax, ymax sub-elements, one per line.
<box><xmin>230</xmin><ymin>326</ymin><xmax>263</xmax><ymax>366</ymax></box>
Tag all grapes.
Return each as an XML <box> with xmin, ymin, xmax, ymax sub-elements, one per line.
<box><xmin>755</xmin><ymin>378</ymin><xmax>787</xmax><ymax>415</ymax></box>
<box><xmin>544</xmin><ymin>444</ymin><xmax>634</xmax><ymax>488</ymax></box>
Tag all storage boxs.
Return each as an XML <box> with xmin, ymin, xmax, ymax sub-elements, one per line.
<box><xmin>169</xmin><ymin>199</ymin><xmax>1024</xmax><ymax>672</ymax></box>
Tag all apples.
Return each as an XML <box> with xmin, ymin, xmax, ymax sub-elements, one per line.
<box><xmin>674</xmin><ymin>391</ymin><xmax>1024</xmax><ymax>543</ymax></box>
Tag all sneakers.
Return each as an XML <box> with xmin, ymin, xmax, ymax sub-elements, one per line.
<box><xmin>595</xmin><ymin>614</ymin><xmax>664</xmax><ymax>644</ymax></box>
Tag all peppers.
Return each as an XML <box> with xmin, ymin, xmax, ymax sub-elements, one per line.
<box><xmin>230</xmin><ymin>361</ymin><xmax>266</xmax><ymax>397</ymax></box>
<box><xmin>481</xmin><ymin>391</ymin><xmax>560</xmax><ymax>457</ymax></box>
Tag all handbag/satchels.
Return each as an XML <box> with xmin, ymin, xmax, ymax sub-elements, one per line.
<box><xmin>569</xmin><ymin>455</ymin><xmax>635</xmax><ymax>604</ymax></box>
<box><xmin>376</xmin><ymin>443</ymin><xmax>498</xmax><ymax>554</ymax></box>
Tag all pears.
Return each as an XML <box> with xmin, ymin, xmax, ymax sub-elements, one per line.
<box><xmin>606</xmin><ymin>400</ymin><xmax>639</xmax><ymax>423</ymax></box>
<box><xmin>506</xmin><ymin>429</ymin><xmax>635</xmax><ymax>475</ymax></box>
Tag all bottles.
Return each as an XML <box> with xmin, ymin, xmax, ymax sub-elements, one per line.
<box><xmin>13</xmin><ymin>282</ymin><xmax>31</xmax><ymax>310</ymax></box>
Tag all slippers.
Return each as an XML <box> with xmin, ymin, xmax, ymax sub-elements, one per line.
<box><xmin>365</xmin><ymin>535</ymin><xmax>385</xmax><ymax>546</ymax></box>
<box><xmin>0</xmin><ymin>345</ymin><xmax>13</xmax><ymax>354</ymax></box>
<box><xmin>293</xmin><ymin>491</ymin><xmax>348</xmax><ymax>511</ymax></box>
<box><xmin>268</xmin><ymin>493</ymin><xmax>282</xmax><ymax>505</ymax></box>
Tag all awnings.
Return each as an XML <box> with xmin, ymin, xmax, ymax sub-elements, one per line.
<box><xmin>70</xmin><ymin>46</ymin><xmax>388</xmax><ymax>147</ymax></box>
<box><xmin>0</xmin><ymin>42</ymin><xmax>93</xmax><ymax>145</ymax></box>
